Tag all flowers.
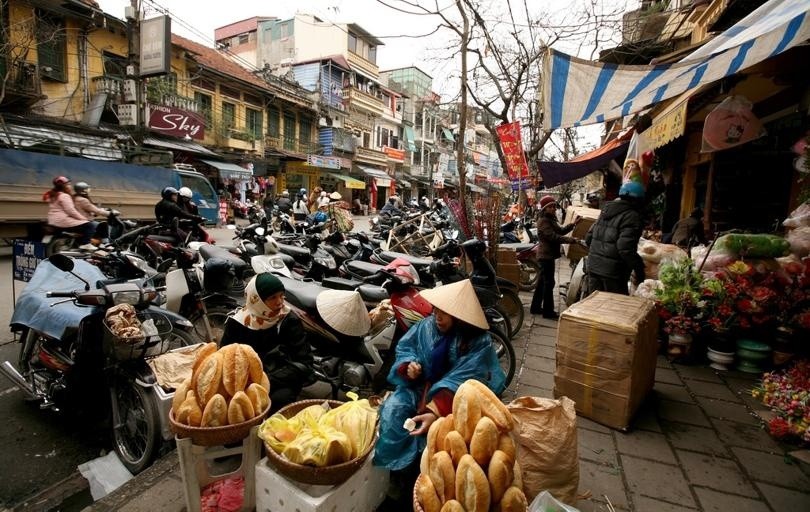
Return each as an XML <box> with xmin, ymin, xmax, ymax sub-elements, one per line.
<box><xmin>651</xmin><ymin>257</ymin><xmax>810</xmax><ymax>442</ymax></box>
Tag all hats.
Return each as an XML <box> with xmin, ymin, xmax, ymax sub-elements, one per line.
<box><xmin>419</xmin><ymin>279</ymin><xmax>490</xmax><ymax>329</ymax></box>
<box><xmin>540</xmin><ymin>195</ymin><xmax>557</xmax><ymax>211</ymax></box>
<box><xmin>330</xmin><ymin>192</ymin><xmax>341</xmax><ymax>199</ymax></box>
<box><xmin>316</xmin><ymin>289</ymin><xmax>371</xmax><ymax>336</ymax></box>
<box><xmin>256</xmin><ymin>272</ymin><xmax>285</xmax><ymax>301</ymax></box>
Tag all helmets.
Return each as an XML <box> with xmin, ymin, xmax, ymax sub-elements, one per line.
<box><xmin>161</xmin><ymin>187</ymin><xmax>193</xmax><ymax>203</ymax></box>
<box><xmin>283</xmin><ymin>192</ymin><xmax>289</xmax><ymax>197</ymax></box>
<box><xmin>300</xmin><ymin>188</ymin><xmax>306</xmax><ymax>193</ymax></box>
<box><xmin>618</xmin><ymin>182</ymin><xmax>646</xmax><ymax>197</ymax></box>
<box><xmin>53</xmin><ymin>176</ymin><xmax>92</xmax><ymax>192</ymax></box>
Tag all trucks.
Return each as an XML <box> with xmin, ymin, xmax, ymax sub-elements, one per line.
<box><xmin>0</xmin><ymin>118</ymin><xmax>221</xmax><ymax>256</ymax></box>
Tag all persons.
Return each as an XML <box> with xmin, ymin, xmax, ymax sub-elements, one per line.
<box><xmin>43</xmin><ymin>176</ymin><xmax>96</xmax><ymax>241</ymax></box>
<box><xmin>373</xmin><ymin>305</ymin><xmax>506</xmax><ymax>471</ymax></box>
<box><xmin>585</xmin><ymin>182</ymin><xmax>645</xmax><ymax>296</ymax></box>
<box><xmin>619</xmin><ymin>159</ymin><xmax>645</xmax><ymax>198</ymax></box>
<box><xmin>530</xmin><ymin>197</ymin><xmax>587</xmax><ymax>319</ymax></box>
<box><xmin>178</xmin><ymin>186</ymin><xmax>198</xmax><ymax>233</ymax></box>
<box><xmin>155</xmin><ymin>187</ymin><xmax>199</xmax><ymax>240</ymax></box>
<box><xmin>379</xmin><ymin>195</ymin><xmax>443</xmax><ymax>217</ymax></box>
<box><xmin>219</xmin><ymin>272</ymin><xmax>317</xmax><ymax>463</ymax></box>
<box><xmin>73</xmin><ymin>181</ymin><xmax>111</xmax><ymax>233</ymax></box>
<box><xmin>262</xmin><ymin>186</ymin><xmax>342</xmax><ymax>233</ymax></box>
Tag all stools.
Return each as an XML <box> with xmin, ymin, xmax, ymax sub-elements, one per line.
<box><xmin>173</xmin><ymin>424</ymin><xmax>263</xmax><ymax>511</ymax></box>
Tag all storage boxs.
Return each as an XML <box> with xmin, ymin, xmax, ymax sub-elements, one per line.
<box><xmin>562</xmin><ymin>206</ymin><xmax>601</xmax><ymax>260</ymax></box>
<box><xmin>496</xmin><ymin>247</ymin><xmax>517</xmax><ymax>264</ymax></box>
<box><xmin>496</xmin><ymin>264</ymin><xmax>520</xmax><ymax>287</ymax></box>
<box><xmin>552</xmin><ymin>290</ymin><xmax>658</xmax><ymax>432</ymax></box>
<box><xmin>496</xmin><ymin>287</ymin><xmax>519</xmax><ymax>316</ymax></box>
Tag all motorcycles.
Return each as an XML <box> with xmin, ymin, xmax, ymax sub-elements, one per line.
<box><xmin>33</xmin><ymin>198</ymin><xmax>540</xmax><ymax>475</ymax></box>
<box><xmin>560</xmin><ymin>240</ymin><xmax>590</xmax><ymax>307</ymax></box>
<box><xmin>0</xmin><ymin>252</ymin><xmax>173</xmax><ymax>479</ymax></box>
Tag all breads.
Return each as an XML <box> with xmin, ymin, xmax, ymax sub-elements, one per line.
<box><xmin>172</xmin><ymin>341</ymin><xmax>271</xmax><ymax>430</ymax></box>
<box><xmin>415</xmin><ymin>379</ymin><xmax>528</xmax><ymax>511</ymax></box>
<box><xmin>265</xmin><ymin>397</ymin><xmax>377</xmax><ymax>466</ymax></box>
